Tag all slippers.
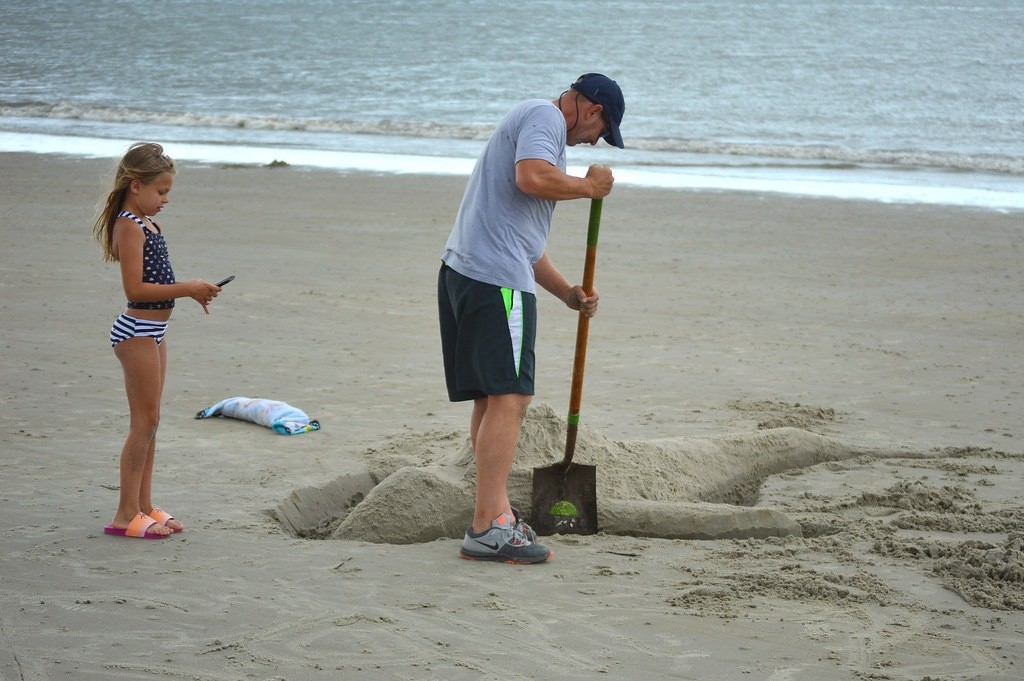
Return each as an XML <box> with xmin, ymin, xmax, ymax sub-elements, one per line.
<box><xmin>104</xmin><ymin>508</ymin><xmax>183</xmax><ymax>538</ymax></box>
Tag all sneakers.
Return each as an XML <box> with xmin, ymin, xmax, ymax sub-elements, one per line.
<box><xmin>458</xmin><ymin>514</ymin><xmax>553</xmax><ymax>565</ymax></box>
<box><xmin>510</xmin><ymin>506</ymin><xmax>537</xmax><ymax>543</ymax></box>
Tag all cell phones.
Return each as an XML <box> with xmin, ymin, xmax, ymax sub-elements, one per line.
<box><xmin>214</xmin><ymin>276</ymin><xmax>235</xmax><ymax>287</ymax></box>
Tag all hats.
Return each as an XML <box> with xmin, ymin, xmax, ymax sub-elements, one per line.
<box><xmin>571</xmin><ymin>73</ymin><xmax>625</xmax><ymax>150</ymax></box>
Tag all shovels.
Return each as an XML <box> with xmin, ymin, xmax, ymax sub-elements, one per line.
<box><xmin>531</xmin><ymin>198</ymin><xmax>603</xmax><ymax>536</ymax></box>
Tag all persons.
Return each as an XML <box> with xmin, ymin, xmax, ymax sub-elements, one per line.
<box><xmin>435</xmin><ymin>74</ymin><xmax>626</xmax><ymax>565</ymax></box>
<box><xmin>91</xmin><ymin>140</ymin><xmax>223</xmax><ymax>538</ymax></box>
